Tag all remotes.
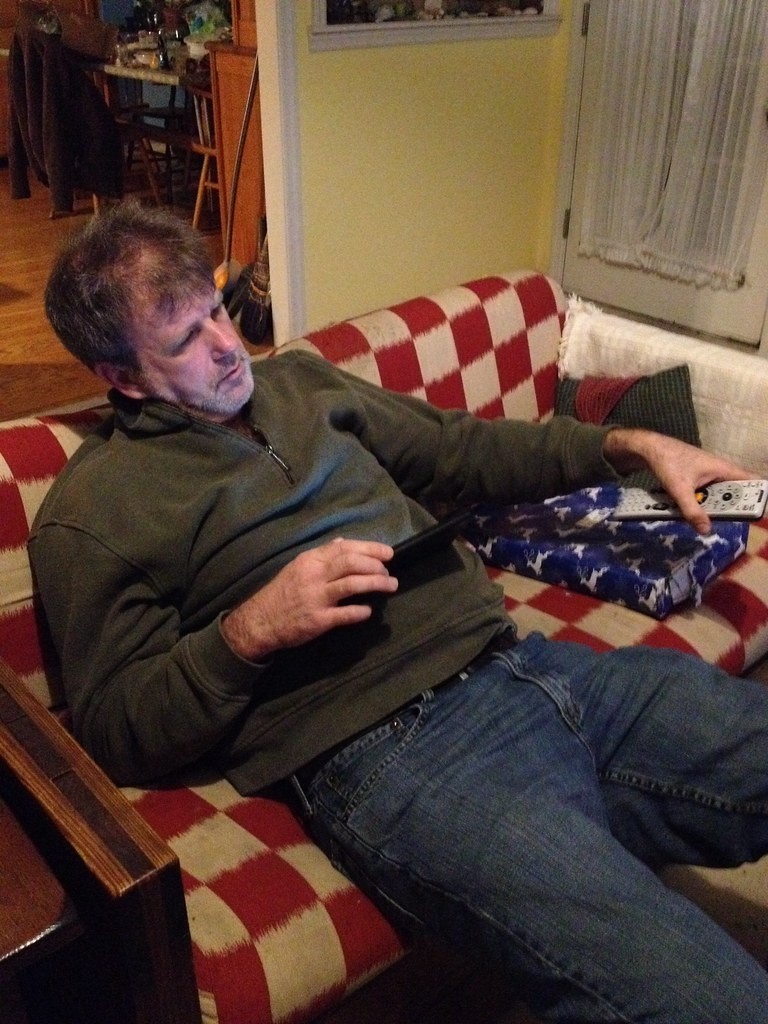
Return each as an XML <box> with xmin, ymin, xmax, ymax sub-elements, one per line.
<box><xmin>611</xmin><ymin>479</ymin><xmax>768</xmax><ymax>521</ymax></box>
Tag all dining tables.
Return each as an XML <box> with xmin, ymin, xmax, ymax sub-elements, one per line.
<box><xmin>0</xmin><ymin>48</ymin><xmax>211</xmax><ymax>87</ymax></box>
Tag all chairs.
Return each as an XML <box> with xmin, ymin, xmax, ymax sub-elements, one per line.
<box><xmin>186</xmin><ymin>84</ymin><xmax>221</xmax><ymax>231</ymax></box>
<box><xmin>17</xmin><ymin>28</ymin><xmax>163</xmax><ymax>221</ymax></box>
<box><xmin>93</xmin><ymin>69</ymin><xmax>191</xmax><ymax>205</ymax></box>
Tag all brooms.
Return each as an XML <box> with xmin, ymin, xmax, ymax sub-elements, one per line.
<box><xmin>238</xmin><ymin>232</ymin><xmax>272</xmax><ymax>346</ymax></box>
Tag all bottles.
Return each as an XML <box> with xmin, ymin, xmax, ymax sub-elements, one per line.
<box><xmin>115</xmin><ymin>38</ymin><xmax>128</xmax><ymax>68</ymax></box>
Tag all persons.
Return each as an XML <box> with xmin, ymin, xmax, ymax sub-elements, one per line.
<box><xmin>26</xmin><ymin>196</ymin><xmax>768</xmax><ymax>1024</ymax></box>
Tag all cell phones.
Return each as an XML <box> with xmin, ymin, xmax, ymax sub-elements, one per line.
<box><xmin>379</xmin><ymin>501</ymin><xmax>482</xmax><ymax>573</ymax></box>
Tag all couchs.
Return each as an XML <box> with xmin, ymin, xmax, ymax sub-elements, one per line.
<box><xmin>1</xmin><ymin>269</ymin><xmax>768</xmax><ymax>1024</ymax></box>
